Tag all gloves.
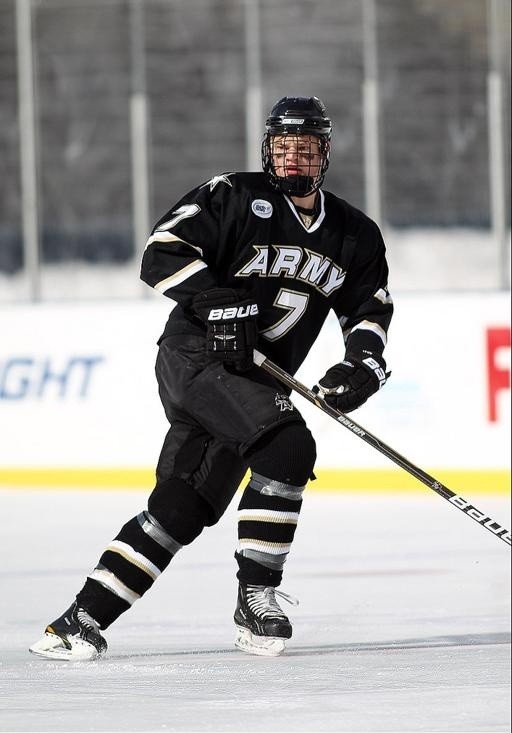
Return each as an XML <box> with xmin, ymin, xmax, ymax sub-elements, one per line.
<box><xmin>197</xmin><ymin>295</ymin><xmax>259</xmax><ymax>364</ymax></box>
<box><xmin>313</xmin><ymin>352</ymin><xmax>388</xmax><ymax>415</ymax></box>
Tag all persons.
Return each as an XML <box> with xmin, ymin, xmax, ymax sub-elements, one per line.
<box><xmin>43</xmin><ymin>95</ymin><xmax>394</xmax><ymax>656</ymax></box>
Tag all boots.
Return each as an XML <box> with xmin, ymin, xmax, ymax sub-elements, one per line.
<box><xmin>235</xmin><ymin>573</ymin><xmax>292</xmax><ymax>639</ymax></box>
<box><xmin>28</xmin><ymin>599</ymin><xmax>106</xmax><ymax>651</ymax></box>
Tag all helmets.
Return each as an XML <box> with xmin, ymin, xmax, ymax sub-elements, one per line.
<box><xmin>261</xmin><ymin>96</ymin><xmax>331</xmax><ymax>196</ymax></box>
